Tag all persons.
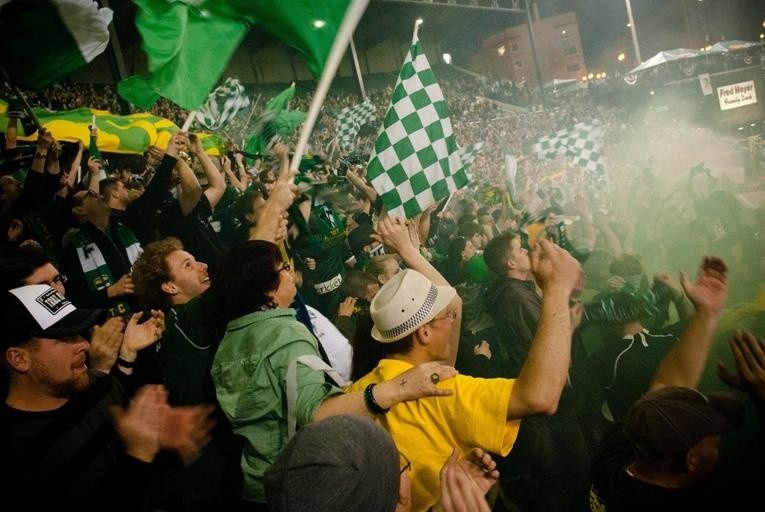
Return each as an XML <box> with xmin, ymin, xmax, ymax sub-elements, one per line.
<box><xmin>0</xmin><ymin>36</ymin><xmax>765</xmax><ymax>512</ymax></box>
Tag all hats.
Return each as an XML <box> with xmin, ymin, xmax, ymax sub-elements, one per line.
<box><xmin>625</xmin><ymin>387</ymin><xmax>745</xmax><ymax>449</ymax></box>
<box><xmin>262</xmin><ymin>415</ymin><xmax>400</xmax><ymax>512</ymax></box>
<box><xmin>369</xmin><ymin>269</ymin><xmax>456</xmax><ymax>343</ymax></box>
<box><xmin>0</xmin><ymin>284</ymin><xmax>102</xmax><ymax>349</ymax></box>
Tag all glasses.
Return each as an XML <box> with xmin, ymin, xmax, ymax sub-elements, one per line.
<box><xmin>80</xmin><ymin>189</ymin><xmax>97</xmax><ymax>200</ymax></box>
<box><xmin>278</xmin><ymin>262</ymin><xmax>291</xmax><ymax>272</ymax></box>
<box><xmin>431</xmin><ymin>310</ymin><xmax>456</xmax><ymax>321</ymax></box>
<box><xmin>45</xmin><ymin>274</ymin><xmax>69</xmax><ymax>287</ymax></box>
<box><xmin>400</xmin><ymin>452</ymin><xmax>411</xmax><ymax>475</ymax></box>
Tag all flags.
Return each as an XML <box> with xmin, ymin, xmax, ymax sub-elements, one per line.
<box><xmin>0</xmin><ymin>0</ymin><xmax>472</xmax><ymax>113</ymax></box>
